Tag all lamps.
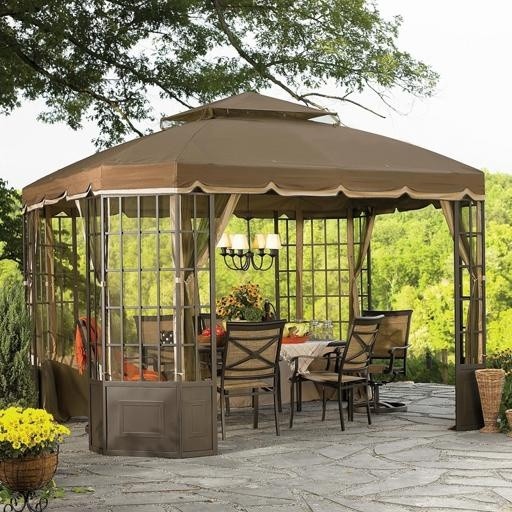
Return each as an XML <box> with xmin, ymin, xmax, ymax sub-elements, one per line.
<box><xmin>217</xmin><ymin>193</ymin><xmax>285</xmax><ymax>273</ymax></box>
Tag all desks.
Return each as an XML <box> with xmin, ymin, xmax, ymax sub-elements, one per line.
<box><xmin>215</xmin><ymin>338</ymin><xmax>348</xmax><ymax>410</ymax></box>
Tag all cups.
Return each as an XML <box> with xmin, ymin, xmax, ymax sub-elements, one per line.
<box><xmin>309</xmin><ymin>320</ymin><xmax>333</xmax><ymax>341</ymax></box>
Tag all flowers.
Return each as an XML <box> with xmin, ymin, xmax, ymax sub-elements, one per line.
<box><xmin>0</xmin><ymin>404</ymin><xmax>69</xmax><ymax>458</ymax></box>
<box><xmin>213</xmin><ymin>282</ymin><xmax>268</xmax><ymax>320</ymax></box>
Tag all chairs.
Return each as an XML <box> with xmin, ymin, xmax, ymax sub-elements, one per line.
<box><xmin>132</xmin><ymin>314</ymin><xmax>174</xmax><ymax>371</ymax></box>
<box><xmin>204</xmin><ymin>319</ymin><xmax>286</xmax><ymax>441</ymax></box>
<box><xmin>76</xmin><ymin>317</ymin><xmax>164</xmax><ymax>383</ymax></box>
<box><xmin>347</xmin><ymin>307</ymin><xmax>413</xmax><ymax>414</ymax></box>
<box><xmin>291</xmin><ymin>314</ymin><xmax>385</xmax><ymax>432</ymax></box>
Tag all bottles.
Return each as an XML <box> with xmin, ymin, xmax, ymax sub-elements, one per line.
<box><xmin>160</xmin><ymin>362</ymin><xmax>167</xmax><ymax>381</ymax></box>
<box><xmin>264</xmin><ymin>300</ymin><xmax>270</xmax><ymax>321</ymax></box>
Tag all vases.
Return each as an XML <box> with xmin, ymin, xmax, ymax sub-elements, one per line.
<box><xmin>0</xmin><ymin>451</ymin><xmax>59</xmax><ymax>512</ymax></box>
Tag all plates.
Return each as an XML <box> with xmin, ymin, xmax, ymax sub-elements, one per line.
<box><xmin>281</xmin><ymin>336</ymin><xmax>308</xmax><ymax>343</ymax></box>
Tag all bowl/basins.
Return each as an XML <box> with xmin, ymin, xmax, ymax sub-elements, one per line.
<box><xmin>196</xmin><ymin>335</ymin><xmax>227</xmax><ymax>347</ymax></box>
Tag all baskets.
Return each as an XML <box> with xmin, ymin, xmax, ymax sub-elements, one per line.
<box><xmin>196</xmin><ymin>322</ymin><xmax>226</xmax><ymax>347</ymax></box>
<box><xmin>474</xmin><ymin>368</ymin><xmax>505</xmax><ymax>433</ymax></box>
<box><xmin>504</xmin><ymin>409</ymin><xmax>512</xmax><ymax>438</ymax></box>
